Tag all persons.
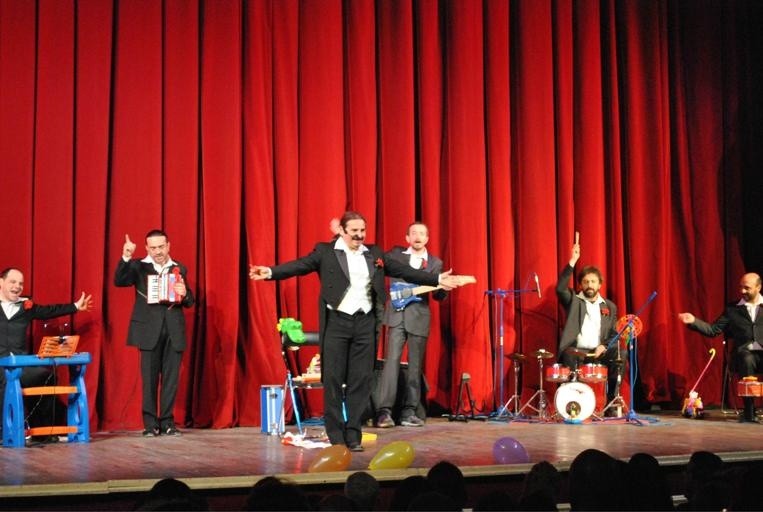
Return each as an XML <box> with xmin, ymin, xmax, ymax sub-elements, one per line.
<box><xmin>556</xmin><ymin>244</ymin><xmax>621</xmax><ymax>416</ymax></box>
<box><xmin>249</xmin><ymin>212</ymin><xmax>460</xmax><ymax>452</ymax></box>
<box><xmin>0</xmin><ymin>268</ymin><xmax>93</xmax><ymax>441</ymax></box>
<box><xmin>141</xmin><ymin>450</ymin><xmax>762</xmax><ymax>512</ymax></box>
<box><xmin>114</xmin><ymin>229</ymin><xmax>195</xmax><ymax>437</ymax></box>
<box><xmin>677</xmin><ymin>272</ymin><xmax>763</xmax><ymax>423</ymax></box>
<box><xmin>329</xmin><ymin>216</ymin><xmax>443</xmax><ymax>428</ymax></box>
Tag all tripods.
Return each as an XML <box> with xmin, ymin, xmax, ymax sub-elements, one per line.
<box><xmin>512</xmin><ymin>360</ymin><xmax>559</xmax><ymax>423</ymax></box>
<box><xmin>598</xmin><ymin>339</ymin><xmax>634</xmax><ymax>420</ymax></box>
<box><xmin>593</xmin><ymin>326</ymin><xmax>660</xmax><ymax>427</ymax></box>
<box><xmin>495</xmin><ymin>362</ymin><xmax>524</xmax><ymax>423</ymax></box>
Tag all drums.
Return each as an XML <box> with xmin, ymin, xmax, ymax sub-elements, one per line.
<box><xmin>737</xmin><ymin>380</ymin><xmax>763</xmax><ymax>397</ymax></box>
<box><xmin>546</xmin><ymin>364</ymin><xmax>570</xmax><ymax>382</ymax></box>
<box><xmin>554</xmin><ymin>381</ymin><xmax>596</xmax><ymax>421</ymax></box>
<box><xmin>577</xmin><ymin>363</ymin><xmax>608</xmax><ymax>384</ymax></box>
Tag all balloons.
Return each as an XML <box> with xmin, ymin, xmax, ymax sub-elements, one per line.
<box><xmin>493</xmin><ymin>438</ymin><xmax>529</xmax><ymax>463</ymax></box>
<box><xmin>308</xmin><ymin>444</ymin><xmax>351</xmax><ymax>471</ymax></box>
<box><xmin>368</xmin><ymin>441</ymin><xmax>414</xmax><ymax>469</ymax></box>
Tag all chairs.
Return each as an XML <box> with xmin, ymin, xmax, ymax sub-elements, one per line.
<box><xmin>275</xmin><ymin>330</ymin><xmax>348</xmax><ymax>438</ymax></box>
<box><xmin>720</xmin><ymin>332</ymin><xmax>763</xmax><ymax>415</ymax></box>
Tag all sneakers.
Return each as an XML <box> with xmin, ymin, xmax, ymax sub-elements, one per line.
<box><xmin>142</xmin><ymin>427</ymin><xmax>160</xmax><ymax>437</ymax></box>
<box><xmin>401</xmin><ymin>415</ymin><xmax>424</xmax><ymax>427</ymax></box>
<box><xmin>161</xmin><ymin>424</ymin><xmax>183</xmax><ymax>436</ymax></box>
<box><xmin>377</xmin><ymin>414</ymin><xmax>396</xmax><ymax>428</ymax></box>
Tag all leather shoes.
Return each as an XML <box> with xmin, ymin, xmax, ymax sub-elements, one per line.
<box><xmin>348</xmin><ymin>442</ymin><xmax>363</xmax><ymax>451</ymax></box>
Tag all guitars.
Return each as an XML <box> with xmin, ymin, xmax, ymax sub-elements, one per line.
<box><xmin>389</xmin><ymin>275</ymin><xmax>476</xmax><ymax>311</ymax></box>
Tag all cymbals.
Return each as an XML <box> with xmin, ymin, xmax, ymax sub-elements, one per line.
<box><xmin>504</xmin><ymin>352</ymin><xmax>527</xmax><ymax>360</ymax></box>
<box><xmin>566</xmin><ymin>347</ymin><xmax>586</xmax><ymax>357</ymax></box>
<box><xmin>532</xmin><ymin>349</ymin><xmax>554</xmax><ymax>359</ymax></box>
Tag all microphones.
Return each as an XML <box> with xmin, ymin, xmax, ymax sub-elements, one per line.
<box><xmin>645</xmin><ymin>291</ymin><xmax>658</xmax><ymax>305</ymax></box>
<box><xmin>530</xmin><ymin>271</ymin><xmax>542</xmax><ymax>299</ymax></box>
